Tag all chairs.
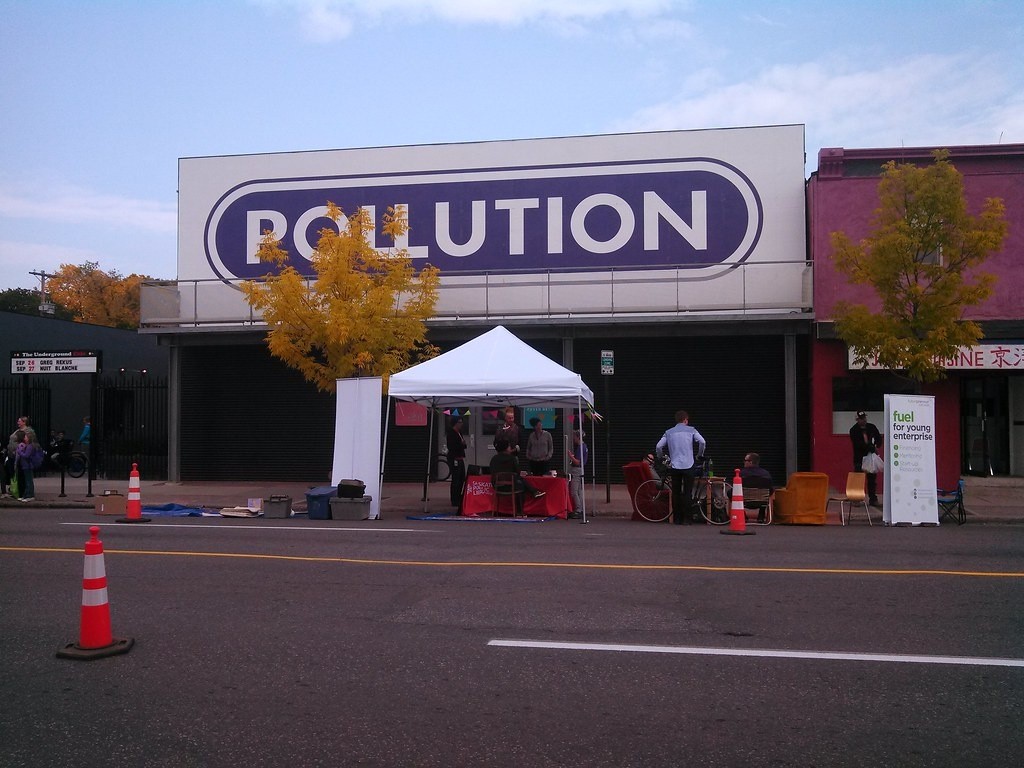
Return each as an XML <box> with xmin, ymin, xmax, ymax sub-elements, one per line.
<box><xmin>825</xmin><ymin>472</ymin><xmax>873</xmax><ymax>526</ymax></box>
<box><xmin>622</xmin><ymin>460</ymin><xmax>672</xmax><ymax>521</ymax></box>
<box><xmin>492</xmin><ymin>471</ymin><xmax>527</xmax><ymax>518</ymax></box>
<box><xmin>742</xmin><ymin>488</ymin><xmax>772</xmax><ymax>527</ymax></box>
<box><xmin>771</xmin><ymin>472</ymin><xmax>829</xmax><ymax>525</ymax></box>
<box><xmin>937</xmin><ymin>479</ymin><xmax>966</xmax><ymax>526</ymax></box>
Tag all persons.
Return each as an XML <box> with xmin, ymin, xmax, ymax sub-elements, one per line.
<box><xmin>446</xmin><ymin>416</ymin><xmax>467</xmax><ymax>507</ymax></box>
<box><xmin>656</xmin><ymin>410</ymin><xmax>706</xmax><ymax>526</ymax></box>
<box><xmin>51</xmin><ymin>428</ymin><xmax>66</xmax><ymax>473</ymax></box>
<box><xmin>78</xmin><ymin>416</ymin><xmax>90</xmax><ymax>468</ymax></box>
<box><xmin>493</xmin><ymin>410</ymin><xmax>525</xmax><ymax>465</ymax></box>
<box><xmin>0</xmin><ymin>415</ymin><xmax>40</xmax><ymax>503</ymax></box>
<box><xmin>727</xmin><ymin>452</ymin><xmax>774</xmax><ymax>522</ymax></box>
<box><xmin>642</xmin><ymin>453</ymin><xmax>673</xmax><ymax>524</ymax></box>
<box><xmin>568</xmin><ymin>429</ymin><xmax>588</xmax><ymax>519</ymax></box>
<box><xmin>848</xmin><ymin>410</ymin><xmax>882</xmax><ymax>507</ymax></box>
<box><xmin>525</xmin><ymin>418</ymin><xmax>553</xmax><ymax>476</ymax></box>
<box><xmin>488</xmin><ymin>439</ymin><xmax>546</xmax><ymax>519</ymax></box>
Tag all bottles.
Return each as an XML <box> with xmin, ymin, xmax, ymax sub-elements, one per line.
<box><xmin>708</xmin><ymin>458</ymin><xmax>714</xmax><ymax>477</ymax></box>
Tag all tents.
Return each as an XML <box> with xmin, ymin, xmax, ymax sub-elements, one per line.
<box><xmin>379</xmin><ymin>325</ymin><xmax>594</xmax><ymax>524</ymax></box>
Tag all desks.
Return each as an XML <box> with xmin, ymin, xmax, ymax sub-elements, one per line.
<box><xmin>462</xmin><ymin>474</ymin><xmax>573</xmax><ymax>518</ymax></box>
<box><xmin>694</xmin><ymin>476</ymin><xmax>726</xmax><ymax>524</ymax></box>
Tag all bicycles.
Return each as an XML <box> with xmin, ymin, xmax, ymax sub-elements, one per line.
<box><xmin>634</xmin><ymin>455</ymin><xmax>734</xmax><ymax>526</ymax></box>
<box><xmin>66</xmin><ymin>441</ymin><xmax>105</xmax><ymax>478</ymax></box>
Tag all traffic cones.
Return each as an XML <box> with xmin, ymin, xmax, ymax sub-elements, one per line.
<box><xmin>115</xmin><ymin>463</ymin><xmax>153</xmax><ymax>524</ymax></box>
<box><xmin>719</xmin><ymin>468</ymin><xmax>757</xmax><ymax>536</ymax></box>
<box><xmin>55</xmin><ymin>525</ymin><xmax>136</xmax><ymax>660</ymax></box>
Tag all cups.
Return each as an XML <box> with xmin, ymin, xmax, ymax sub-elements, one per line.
<box><xmin>549</xmin><ymin>470</ymin><xmax>557</xmax><ymax>478</ymax></box>
<box><xmin>520</xmin><ymin>470</ymin><xmax>526</xmax><ymax>477</ymax></box>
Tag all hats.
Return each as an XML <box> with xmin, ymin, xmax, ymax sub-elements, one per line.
<box><xmin>573</xmin><ymin>429</ymin><xmax>586</xmax><ymax>438</ymax></box>
<box><xmin>856</xmin><ymin>409</ymin><xmax>867</xmax><ymax>418</ymax></box>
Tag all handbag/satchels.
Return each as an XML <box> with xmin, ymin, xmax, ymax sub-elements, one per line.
<box><xmin>861</xmin><ymin>452</ymin><xmax>884</xmax><ymax>473</ymax></box>
<box><xmin>11</xmin><ymin>473</ymin><xmax>19</xmax><ymax>497</ymax></box>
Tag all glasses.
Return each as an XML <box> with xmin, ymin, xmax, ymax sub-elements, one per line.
<box><xmin>744</xmin><ymin>459</ymin><xmax>752</xmax><ymax>462</ymax></box>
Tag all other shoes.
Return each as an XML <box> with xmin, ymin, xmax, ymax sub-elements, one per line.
<box><xmin>534</xmin><ymin>490</ymin><xmax>547</xmax><ymax>498</ymax></box>
<box><xmin>870</xmin><ymin>501</ymin><xmax>880</xmax><ymax>506</ymax></box>
<box><xmin>22</xmin><ymin>498</ymin><xmax>35</xmax><ymax>502</ymax></box>
<box><xmin>18</xmin><ymin>497</ymin><xmax>24</xmax><ymax>501</ymax></box>
<box><xmin>573</xmin><ymin>511</ymin><xmax>582</xmax><ymax>519</ymax></box>
<box><xmin>516</xmin><ymin>514</ymin><xmax>527</xmax><ymax>518</ymax></box>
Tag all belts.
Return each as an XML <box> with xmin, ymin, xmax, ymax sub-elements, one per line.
<box><xmin>572</xmin><ymin>465</ymin><xmax>581</xmax><ymax>467</ymax></box>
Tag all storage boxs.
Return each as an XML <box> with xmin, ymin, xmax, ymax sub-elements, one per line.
<box><xmin>94</xmin><ymin>494</ymin><xmax>126</xmax><ymax>516</ymax></box>
<box><xmin>305</xmin><ymin>486</ymin><xmax>338</xmax><ymax>520</ymax></box>
<box><xmin>330</xmin><ymin>495</ymin><xmax>373</xmax><ymax>520</ymax></box>
<box><xmin>262</xmin><ymin>498</ymin><xmax>292</xmax><ymax>516</ymax></box>
